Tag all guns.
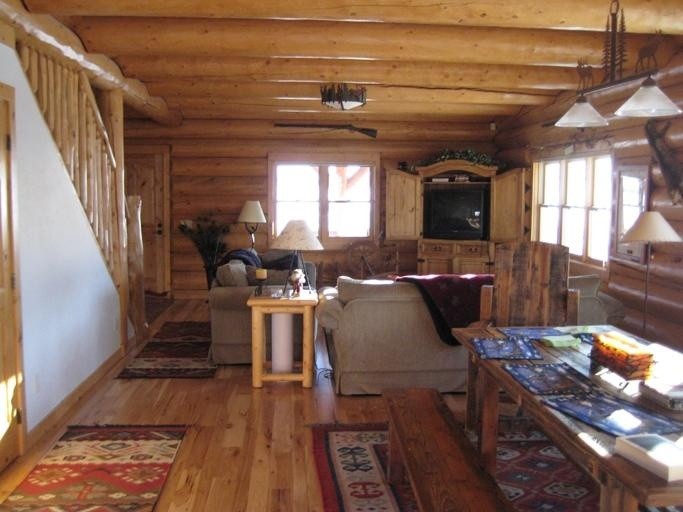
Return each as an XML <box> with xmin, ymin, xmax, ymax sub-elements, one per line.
<box><xmin>273</xmin><ymin>121</ymin><xmax>376</xmax><ymax>138</ymax></box>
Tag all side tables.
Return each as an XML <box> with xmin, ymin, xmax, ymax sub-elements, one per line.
<box><xmin>246</xmin><ymin>290</ymin><xmax>318</xmax><ymax>389</ymax></box>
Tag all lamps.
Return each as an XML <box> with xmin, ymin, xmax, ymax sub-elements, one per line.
<box><xmin>554</xmin><ymin>1</ymin><xmax>683</xmax><ymax>129</ymax></box>
<box><xmin>270</xmin><ymin>220</ymin><xmax>325</xmax><ymax>295</ymax></box>
<box><xmin>237</xmin><ymin>199</ymin><xmax>268</xmax><ymax>248</ymax></box>
<box><xmin>319</xmin><ymin>84</ymin><xmax>367</xmax><ymax>112</ymax></box>
<box><xmin>619</xmin><ymin>211</ymin><xmax>683</xmax><ymax>337</ymax></box>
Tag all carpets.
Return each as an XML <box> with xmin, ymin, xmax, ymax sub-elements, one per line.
<box><xmin>0</xmin><ymin>424</ymin><xmax>186</xmax><ymax>512</ymax></box>
<box><xmin>118</xmin><ymin>321</ymin><xmax>218</xmax><ymax>379</ymax></box>
<box><xmin>312</xmin><ymin>414</ymin><xmax>600</xmax><ymax>512</ymax></box>
<box><xmin>144</xmin><ymin>294</ymin><xmax>176</xmax><ymax>325</ymax></box>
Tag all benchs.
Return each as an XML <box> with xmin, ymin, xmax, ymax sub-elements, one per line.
<box><xmin>383</xmin><ymin>388</ymin><xmax>515</xmax><ymax>512</ymax></box>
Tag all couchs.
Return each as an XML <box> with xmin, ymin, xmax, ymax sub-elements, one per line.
<box><xmin>208</xmin><ymin>252</ymin><xmax>316</xmax><ymax>366</ymax></box>
<box><xmin>316</xmin><ymin>275</ymin><xmax>624</xmax><ymax>396</ymax></box>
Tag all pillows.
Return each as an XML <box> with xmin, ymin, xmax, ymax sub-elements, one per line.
<box><xmin>246</xmin><ymin>264</ymin><xmax>305</xmax><ymax>286</ymax></box>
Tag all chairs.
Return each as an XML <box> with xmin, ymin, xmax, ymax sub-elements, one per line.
<box><xmin>467</xmin><ymin>241</ymin><xmax>579</xmax><ymax>426</ymax></box>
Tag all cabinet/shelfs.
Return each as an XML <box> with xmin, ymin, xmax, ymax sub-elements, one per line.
<box><xmin>385</xmin><ymin>160</ymin><xmax>525</xmax><ymax>275</ymax></box>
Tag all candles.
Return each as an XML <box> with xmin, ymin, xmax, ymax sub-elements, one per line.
<box><xmin>256</xmin><ymin>267</ymin><xmax>268</xmax><ymax>280</ymax></box>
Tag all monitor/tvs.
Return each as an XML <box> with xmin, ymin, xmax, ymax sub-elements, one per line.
<box><xmin>430</xmin><ymin>188</ymin><xmax>484</xmax><ymax>240</ymax></box>
